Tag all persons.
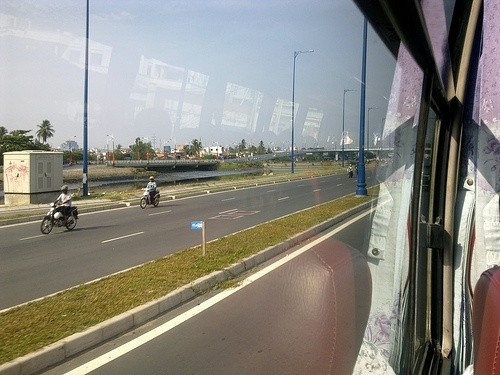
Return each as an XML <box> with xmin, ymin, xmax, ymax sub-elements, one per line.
<box><xmin>52</xmin><ymin>185</ymin><xmax>72</xmax><ymax>227</ymax></box>
<box><xmin>347</xmin><ymin>163</ymin><xmax>358</xmax><ymax>178</ymax></box>
<box><xmin>145</xmin><ymin>176</ymin><xmax>157</xmax><ymax>204</ymax></box>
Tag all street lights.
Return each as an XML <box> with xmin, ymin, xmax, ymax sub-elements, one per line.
<box><xmin>71</xmin><ymin>136</ymin><xmax>76</xmax><ymax>161</ymax></box>
<box><xmin>170</xmin><ymin>138</ymin><xmax>176</xmax><ymax>161</ymax></box>
<box><xmin>368</xmin><ymin>107</ymin><xmax>376</xmax><ymax>163</ymax></box>
<box><xmin>291</xmin><ymin>50</ymin><xmax>315</xmax><ymax>173</ymax></box>
<box><xmin>107</xmin><ymin>134</ymin><xmax>114</xmax><ymax>165</ymax></box>
<box><xmin>343</xmin><ymin>89</ymin><xmax>359</xmax><ymax>167</ymax></box>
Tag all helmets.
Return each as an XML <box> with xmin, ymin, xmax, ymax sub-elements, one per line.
<box><xmin>61</xmin><ymin>185</ymin><xmax>68</xmax><ymax>194</ymax></box>
<box><xmin>149</xmin><ymin>177</ymin><xmax>154</xmax><ymax>181</ymax></box>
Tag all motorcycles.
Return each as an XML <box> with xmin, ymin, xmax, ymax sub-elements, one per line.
<box><xmin>140</xmin><ymin>188</ymin><xmax>160</xmax><ymax>209</ymax></box>
<box><xmin>348</xmin><ymin>169</ymin><xmax>353</xmax><ymax>178</ymax></box>
<box><xmin>40</xmin><ymin>203</ymin><xmax>79</xmax><ymax>233</ymax></box>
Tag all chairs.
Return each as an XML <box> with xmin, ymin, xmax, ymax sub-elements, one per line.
<box><xmin>245</xmin><ymin>231</ymin><xmax>372</xmax><ymax>375</ymax></box>
<box><xmin>473</xmin><ymin>266</ymin><xmax>500</xmax><ymax>375</ymax></box>
